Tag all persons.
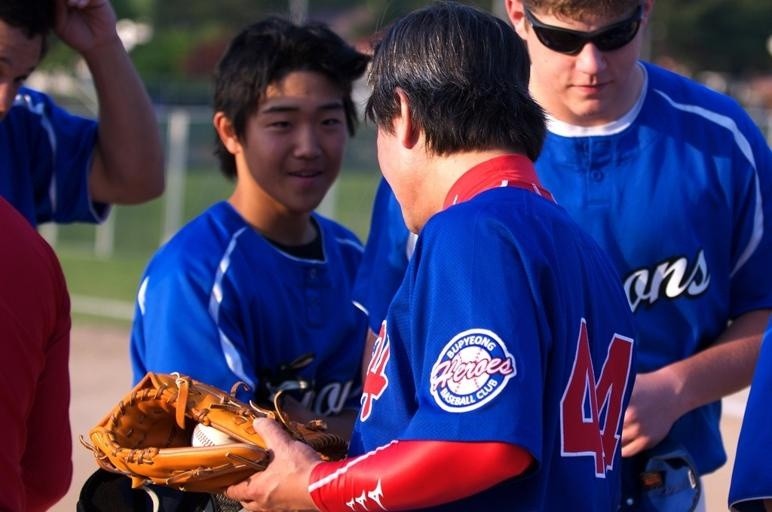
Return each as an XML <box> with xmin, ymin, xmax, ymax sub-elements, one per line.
<box><xmin>354</xmin><ymin>0</ymin><xmax>772</xmax><ymax>511</ymax></box>
<box><xmin>129</xmin><ymin>16</ymin><xmax>372</xmax><ymax>455</ymax></box>
<box><xmin>225</xmin><ymin>0</ymin><xmax>638</xmax><ymax>511</ymax></box>
<box><xmin>1</xmin><ymin>195</ymin><xmax>74</xmax><ymax>512</ymax></box>
<box><xmin>0</xmin><ymin>1</ymin><xmax>165</xmax><ymax>230</ymax></box>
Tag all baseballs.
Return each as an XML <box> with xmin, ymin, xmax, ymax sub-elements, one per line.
<box><xmin>192</xmin><ymin>422</ymin><xmax>232</xmax><ymax>446</ymax></box>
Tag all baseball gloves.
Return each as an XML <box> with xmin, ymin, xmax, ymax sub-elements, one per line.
<box><xmin>79</xmin><ymin>372</ymin><xmax>349</xmax><ymax>493</ymax></box>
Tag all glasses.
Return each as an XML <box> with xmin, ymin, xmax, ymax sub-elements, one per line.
<box><xmin>526</xmin><ymin>6</ymin><xmax>642</xmax><ymax>51</ymax></box>
